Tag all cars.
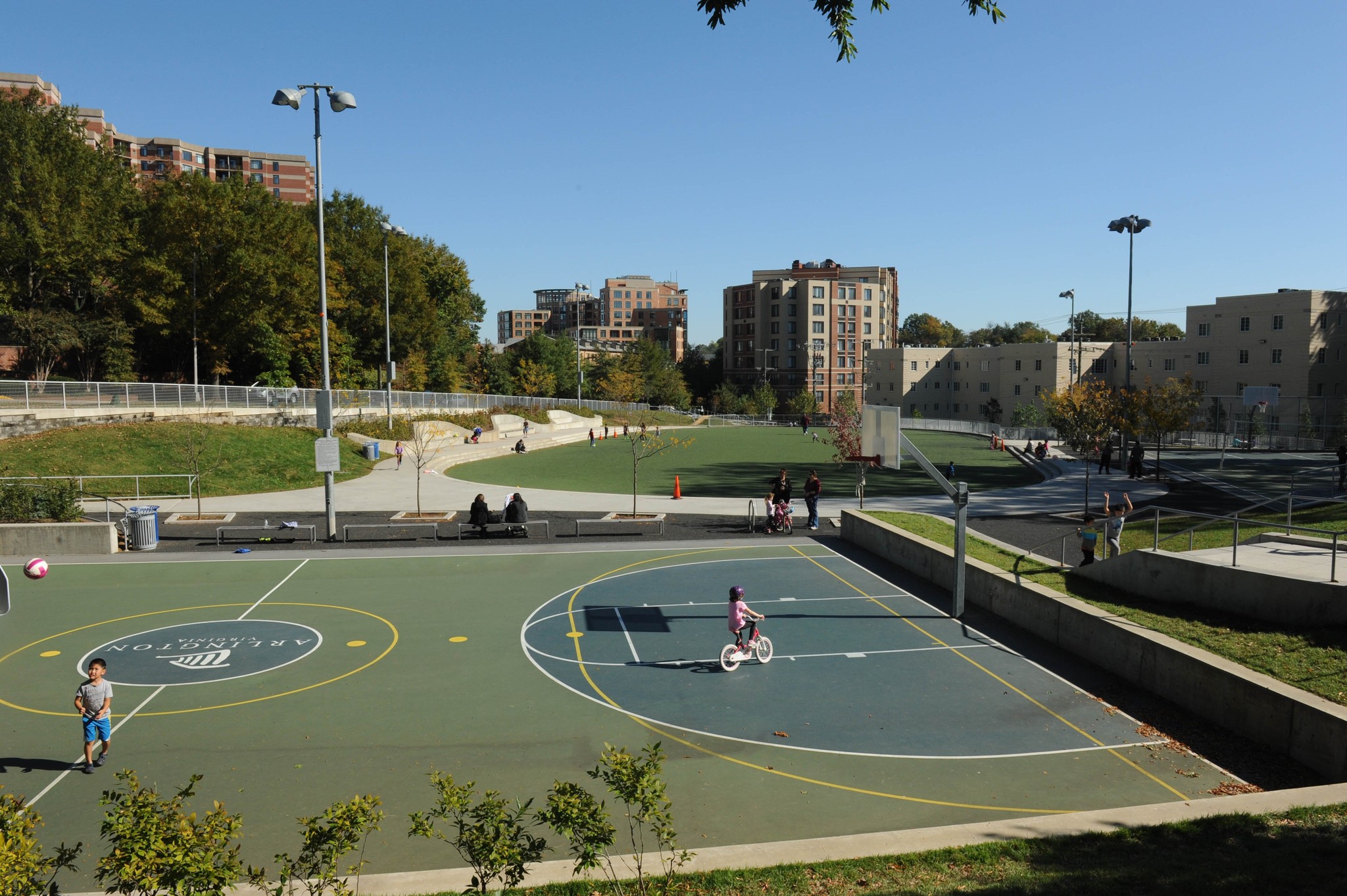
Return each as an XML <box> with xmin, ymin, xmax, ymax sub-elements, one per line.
<box><xmin>241</xmin><ymin>380</ymin><xmax>300</xmax><ymax>404</ymax></box>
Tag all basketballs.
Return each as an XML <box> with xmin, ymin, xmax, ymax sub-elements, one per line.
<box><xmin>23</xmin><ymin>558</ymin><xmax>48</xmax><ymax>579</ymax></box>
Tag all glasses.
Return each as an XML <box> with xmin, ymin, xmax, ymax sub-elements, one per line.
<box><xmin>809</xmin><ymin>474</ymin><xmax>813</xmax><ymax>476</ymax></box>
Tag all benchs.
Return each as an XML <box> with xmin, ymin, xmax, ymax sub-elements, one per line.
<box><xmin>458</xmin><ymin>520</ymin><xmax>549</xmax><ymax>541</ymax></box>
<box><xmin>343</xmin><ymin>523</ymin><xmax>437</xmax><ymax>544</ymax></box>
<box><xmin>216</xmin><ymin>525</ymin><xmax>316</xmax><ymax>544</ymax></box>
<box><xmin>576</xmin><ymin>518</ymin><xmax>664</xmax><ymax>537</ymax></box>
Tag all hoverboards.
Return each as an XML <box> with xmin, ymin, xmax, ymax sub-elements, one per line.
<box><xmin>395</xmin><ymin>454</ymin><xmax>402</xmax><ymax>470</ymax></box>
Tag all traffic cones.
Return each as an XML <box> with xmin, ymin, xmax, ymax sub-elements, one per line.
<box><xmin>670</xmin><ymin>475</ymin><xmax>683</xmax><ymax>500</ymax></box>
<box><xmin>598</xmin><ymin>431</ymin><xmax>604</xmax><ymax>440</ymax></box>
<box><xmin>612</xmin><ymin>427</ymin><xmax>618</xmax><ymax>438</ymax></box>
<box><xmin>993</xmin><ymin>436</ymin><xmax>999</xmax><ymax>448</ymax></box>
<box><xmin>1000</xmin><ymin>438</ymin><xmax>1005</xmax><ymax>452</ymax></box>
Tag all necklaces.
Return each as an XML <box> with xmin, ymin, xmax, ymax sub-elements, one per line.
<box><xmin>780</xmin><ymin>478</ymin><xmax>785</xmax><ymax>485</ymax></box>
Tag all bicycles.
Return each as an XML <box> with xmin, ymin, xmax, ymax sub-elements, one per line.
<box><xmin>719</xmin><ymin>615</ymin><xmax>773</xmax><ymax>671</ymax></box>
<box><xmin>766</xmin><ymin>499</ymin><xmax>795</xmax><ymax>534</ymax></box>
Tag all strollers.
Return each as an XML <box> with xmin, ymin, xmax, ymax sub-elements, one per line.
<box><xmin>501</xmin><ymin>493</ymin><xmax>528</xmax><ymax>537</ymax></box>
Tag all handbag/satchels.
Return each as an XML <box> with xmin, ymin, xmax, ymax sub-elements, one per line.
<box><xmin>486</xmin><ymin>514</ymin><xmax>503</xmax><ymax>524</ymax></box>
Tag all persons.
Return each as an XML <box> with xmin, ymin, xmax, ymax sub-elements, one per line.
<box><xmin>989</xmin><ymin>430</ymin><xmax>996</xmax><ymax>450</ymax></box>
<box><xmin>395</xmin><ymin>441</ymin><xmax>403</xmax><ymax>469</ymax></box>
<box><xmin>803</xmin><ymin>469</ymin><xmax>821</xmax><ymax>530</ymax></box>
<box><xmin>728</xmin><ymin>586</ymin><xmax>765</xmax><ymax>662</ymax></box>
<box><xmin>74</xmin><ymin>658</ymin><xmax>113</xmax><ymax>774</ymax></box>
<box><xmin>473</xmin><ymin>424</ymin><xmax>482</xmax><ymax>444</ymax></box>
<box><xmin>946</xmin><ymin>461</ymin><xmax>955</xmax><ymax>481</ymax></box>
<box><xmin>623</xmin><ymin>422</ymin><xmax>629</xmax><ymax>440</ymax></box>
<box><xmin>639</xmin><ymin>423</ymin><xmax>647</xmax><ymax>442</ymax></box>
<box><xmin>523</xmin><ymin>418</ymin><xmax>529</xmax><ymax>438</ymax></box>
<box><xmin>605</xmin><ymin>424</ymin><xmax>608</xmax><ymax>439</ymax></box>
<box><xmin>1336</xmin><ymin>446</ymin><xmax>1347</xmax><ymax>492</ymax></box>
<box><xmin>656</xmin><ymin>427</ymin><xmax>660</xmax><ymax>441</ymax></box>
<box><xmin>1020</xmin><ymin>437</ymin><xmax>1145</xmax><ymax>569</ymax></box>
<box><xmin>588</xmin><ymin>428</ymin><xmax>595</xmax><ymax>447</ymax></box>
<box><xmin>509</xmin><ymin>493</ymin><xmax>528</xmax><ymax>537</ymax></box>
<box><xmin>1232</xmin><ymin>435</ymin><xmax>1256</xmax><ymax>449</ymax></box>
<box><xmin>515</xmin><ymin>439</ymin><xmax>528</xmax><ymax>453</ymax></box>
<box><xmin>801</xmin><ymin>414</ymin><xmax>809</xmax><ymax>435</ymax></box>
<box><xmin>764</xmin><ymin>468</ymin><xmax>792</xmax><ymax>533</ymax></box>
<box><xmin>469</xmin><ymin>494</ymin><xmax>494</xmax><ymax>535</ymax></box>
<box><xmin>812</xmin><ymin>433</ymin><xmax>821</xmax><ymax>443</ymax></box>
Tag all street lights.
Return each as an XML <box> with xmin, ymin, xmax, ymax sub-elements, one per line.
<box><xmin>1107</xmin><ymin>214</ymin><xmax>1152</xmax><ymax>472</ymax></box>
<box><xmin>1059</xmin><ymin>289</ymin><xmax>1075</xmax><ymax>396</ymax></box>
<box><xmin>191</xmin><ymin>243</ymin><xmax>226</xmax><ymax>402</ymax></box>
<box><xmin>575</xmin><ymin>281</ymin><xmax>591</xmax><ymax>410</ymax></box>
<box><xmin>379</xmin><ymin>222</ymin><xmax>409</xmax><ymax>429</ymax></box>
<box><xmin>272</xmin><ymin>82</ymin><xmax>359</xmax><ymax>541</ymax></box>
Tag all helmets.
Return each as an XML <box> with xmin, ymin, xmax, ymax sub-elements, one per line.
<box><xmin>729</xmin><ymin>586</ymin><xmax>745</xmax><ymax>599</ymax></box>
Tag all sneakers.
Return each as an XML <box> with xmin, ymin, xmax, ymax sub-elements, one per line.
<box><xmin>747</xmin><ymin>640</ymin><xmax>758</xmax><ymax>647</ymax></box>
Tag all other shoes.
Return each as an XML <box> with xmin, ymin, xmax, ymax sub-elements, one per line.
<box><xmin>764</xmin><ymin>528</ymin><xmax>773</xmax><ymax>533</ymax></box>
<box><xmin>83</xmin><ymin>762</ymin><xmax>95</xmax><ymax>774</ymax></box>
<box><xmin>95</xmin><ymin>752</ymin><xmax>108</xmax><ymax>766</ymax></box>
<box><xmin>811</xmin><ymin>526</ymin><xmax>818</xmax><ymax>530</ymax></box>
<box><xmin>400</xmin><ymin>462</ymin><xmax>402</xmax><ymax>465</ymax></box>
<box><xmin>803</xmin><ymin>524</ymin><xmax>810</xmax><ymax>527</ymax></box>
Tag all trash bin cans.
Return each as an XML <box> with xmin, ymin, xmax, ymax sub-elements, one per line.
<box><xmin>127</xmin><ymin>510</ymin><xmax>157</xmax><ymax>550</ymax></box>
<box><xmin>360</xmin><ymin>441</ymin><xmax>380</xmax><ymax>461</ymax></box>
<box><xmin>130</xmin><ymin>506</ymin><xmax>159</xmax><ymax>545</ymax></box>
